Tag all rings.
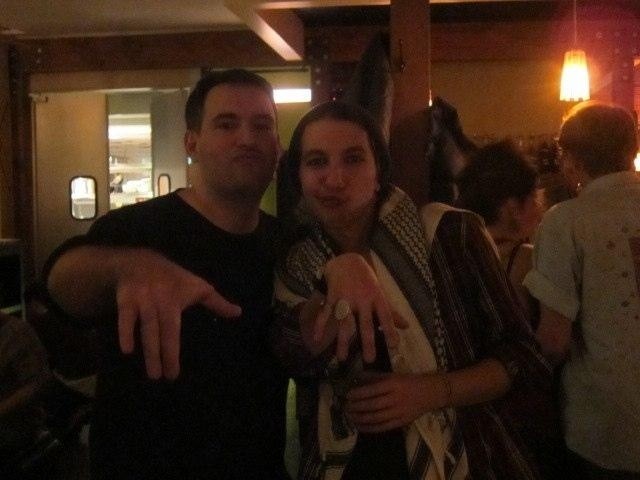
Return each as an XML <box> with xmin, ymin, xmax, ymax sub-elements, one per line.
<box><xmin>334</xmin><ymin>300</ymin><xmax>350</xmax><ymax>318</ymax></box>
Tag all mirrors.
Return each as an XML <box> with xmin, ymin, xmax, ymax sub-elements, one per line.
<box><xmin>69</xmin><ymin>175</ymin><xmax>97</xmax><ymax>221</ymax></box>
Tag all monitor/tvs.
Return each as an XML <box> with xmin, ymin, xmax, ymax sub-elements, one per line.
<box><xmin>0</xmin><ymin>237</ymin><xmax>26</xmax><ymax>314</ymax></box>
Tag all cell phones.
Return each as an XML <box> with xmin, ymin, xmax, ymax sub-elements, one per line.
<box><xmin>337</xmin><ymin>328</ymin><xmax>392</xmax><ymax>380</ymax></box>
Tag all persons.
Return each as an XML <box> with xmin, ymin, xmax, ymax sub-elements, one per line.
<box><xmin>446</xmin><ymin>129</ymin><xmax>543</xmax><ymax>335</ymax></box>
<box><xmin>520</xmin><ymin>100</ymin><xmax>640</xmax><ymax>480</ymax></box>
<box><xmin>41</xmin><ymin>67</ymin><xmax>289</xmax><ymax>479</ymax></box>
<box><xmin>268</xmin><ymin>93</ymin><xmax>560</xmax><ymax>479</ymax></box>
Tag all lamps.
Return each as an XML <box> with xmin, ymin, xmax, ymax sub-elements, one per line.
<box><xmin>558</xmin><ymin>0</ymin><xmax>591</xmax><ymax>103</ymax></box>
